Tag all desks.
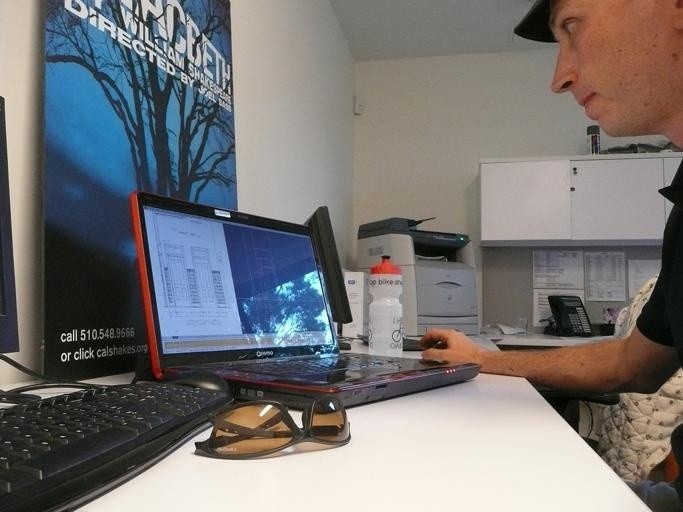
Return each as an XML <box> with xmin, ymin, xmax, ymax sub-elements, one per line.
<box><xmin>489</xmin><ymin>329</ymin><xmax>611</xmax><ymax>347</ymax></box>
<box><xmin>0</xmin><ymin>334</ymin><xmax>653</xmax><ymax>512</ymax></box>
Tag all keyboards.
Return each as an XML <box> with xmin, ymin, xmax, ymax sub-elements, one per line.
<box><xmin>0</xmin><ymin>381</ymin><xmax>236</xmax><ymax>512</ymax></box>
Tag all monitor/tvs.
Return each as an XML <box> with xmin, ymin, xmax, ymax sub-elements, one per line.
<box><xmin>305</xmin><ymin>205</ymin><xmax>354</xmax><ymax>350</ymax></box>
<box><xmin>0</xmin><ymin>96</ymin><xmax>22</xmax><ymax>354</ymax></box>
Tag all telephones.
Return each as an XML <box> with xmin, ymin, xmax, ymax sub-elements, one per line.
<box><xmin>548</xmin><ymin>295</ymin><xmax>592</xmax><ymax>337</ymax></box>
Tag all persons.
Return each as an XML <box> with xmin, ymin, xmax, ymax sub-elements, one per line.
<box><xmin>420</xmin><ymin>0</ymin><xmax>683</xmax><ymax>512</ymax></box>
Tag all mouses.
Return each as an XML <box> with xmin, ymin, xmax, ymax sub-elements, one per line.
<box><xmin>174</xmin><ymin>371</ymin><xmax>232</xmax><ymax>393</ymax></box>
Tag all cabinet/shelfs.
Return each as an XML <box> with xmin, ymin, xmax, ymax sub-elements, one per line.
<box><xmin>664</xmin><ymin>152</ymin><xmax>683</xmax><ymax>231</ymax></box>
<box><xmin>480</xmin><ymin>153</ymin><xmax>664</xmax><ymax>247</ymax></box>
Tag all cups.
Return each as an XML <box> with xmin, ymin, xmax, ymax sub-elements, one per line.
<box><xmin>514</xmin><ymin>312</ymin><xmax>528</xmax><ymax>335</ymax></box>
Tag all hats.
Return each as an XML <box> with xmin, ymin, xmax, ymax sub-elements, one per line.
<box><xmin>510</xmin><ymin>0</ymin><xmax>566</xmax><ymax>43</ymax></box>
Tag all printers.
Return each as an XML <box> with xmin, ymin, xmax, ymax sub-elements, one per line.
<box><xmin>357</xmin><ymin>216</ymin><xmax>480</xmax><ymax>338</ymax></box>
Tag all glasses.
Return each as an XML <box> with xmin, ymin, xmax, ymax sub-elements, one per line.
<box><xmin>191</xmin><ymin>391</ymin><xmax>354</xmax><ymax>463</ymax></box>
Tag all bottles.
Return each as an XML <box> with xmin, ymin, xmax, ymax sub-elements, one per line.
<box><xmin>587</xmin><ymin>125</ymin><xmax>602</xmax><ymax>157</ymax></box>
<box><xmin>366</xmin><ymin>255</ymin><xmax>404</xmax><ymax>356</ymax></box>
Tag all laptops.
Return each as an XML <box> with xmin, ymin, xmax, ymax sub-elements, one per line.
<box><xmin>129</xmin><ymin>189</ymin><xmax>482</xmax><ymax>409</ymax></box>
